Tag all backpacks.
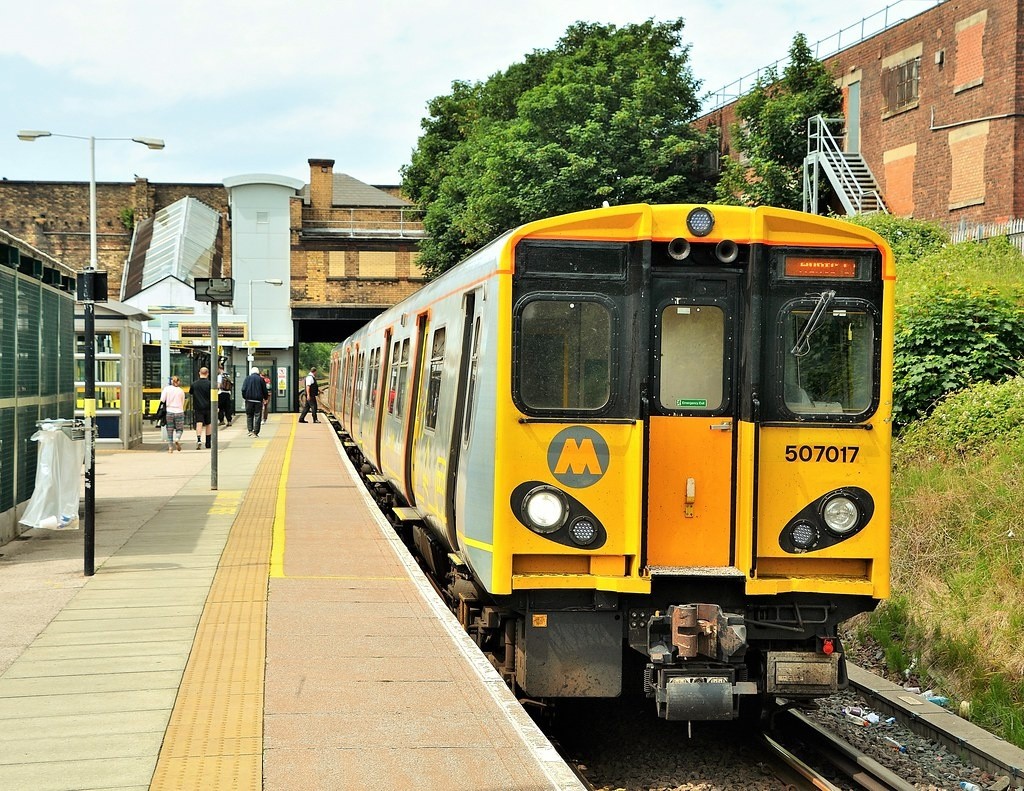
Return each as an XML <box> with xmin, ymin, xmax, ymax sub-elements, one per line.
<box><xmin>220</xmin><ymin>377</ymin><xmax>232</xmax><ymax>391</ymax></box>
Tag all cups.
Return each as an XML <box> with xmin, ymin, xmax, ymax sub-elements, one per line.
<box><xmin>845</xmin><ymin>706</ymin><xmax>862</xmax><ymax>716</ymax></box>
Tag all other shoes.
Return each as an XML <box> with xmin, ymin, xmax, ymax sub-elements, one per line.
<box><xmin>219</xmin><ymin>422</ymin><xmax>225</xmax><ymax>425</ymax></box>
<box><xmin>313</xmin><ymin>420</ymin><xmax>322</xmax><ymax>423</ymax></box>
<box><xmin>226</xmin><ymin>422</ymin><xmax>232</xmax><ymax>426</ymax></box>
<box><xmin>299</xmin><ymin>419</ymin><xmax>308</xmax><ymax>423</ymax></box>
<box><xmin>246</xmin><ymin>431</ymin><xmax>259</xmax><ymax>438</ymax></box>
<box><xmin>261</xmin><ymin>419</ymin><xmax>265</xmax><ymax>425</ymax></box>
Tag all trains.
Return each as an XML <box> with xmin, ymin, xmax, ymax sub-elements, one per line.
<box><xmin>326</xmin><ymin>203</ymin><xmax>891</xmax><ymax>739</ymax></box>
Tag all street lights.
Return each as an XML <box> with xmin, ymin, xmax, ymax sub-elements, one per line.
<box><xmin>249</xmin><ymin>279</ymin><xmax>283</xmax><ymax>375</ymax></box>
<box><xmin>17</xmin><ymin>129</ymin><xmax>165</xmax><ymax>271</ymax></box>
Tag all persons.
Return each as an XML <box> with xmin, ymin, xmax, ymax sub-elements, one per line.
<box><xmin>299</xmin><ymin>367</ymin><xmax>324</xmax><ymax>423</ymax></box>
<box><xmin>260</xmin><ymin>371</ymin><xmax>272</xmax><ymax>424</ymax></box>
<box><xmin>241</xmin><ymin>367</ymin><xmax>268</xmax><ymax>438</ymax></box>
<box><xmin>189</xmin><ymin>367</ymin><xmax>211</xmax><ymax>449</ymax></box>
<box><xmin>161</xmin><ymin>376</ymin><xmax>186</xmax><ymax>453</ymax></box>
<box><xmin>217</xmin><ymin>366</ymin><xmax>234</xmax><ymax>426</ymax></box>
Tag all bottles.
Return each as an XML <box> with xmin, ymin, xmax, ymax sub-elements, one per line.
<box><xmin>960</xmin><ymin>781</ymin><xmax>980</xmax><ymax>791</ymax></box>
<box><xmin>929</xmin><ymin>697</ymin><xmax>950</xmax><ymax>706</ymax></box>
<box><xmin>76</xmin><ymin>392</ymin><xmax>83</xmax><ymax>409</ymax></box>
<box><xmin>846</xmin><ymin>714</ymin><xmax>868</xmax><ymax>726</ymax></box>
<box><xmin>884</xmin><ymin>737</ymin><xmax>905</xmax><ymax>752</ymax></box>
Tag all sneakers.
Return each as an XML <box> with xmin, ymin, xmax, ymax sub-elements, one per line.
<box><xmin>168</xmin><ymin>446</ymin><xmax>173</xmax><ymax>453</ymax></box>
<box><xmin>175</xmin><ymin>441</ymin><xmax>182</xmax><ymax>452</ymax></box>
<box><xmin>196</xmin><ymin>440</ymin><xmax>202</xmax><ymax>450</ymax></box>
